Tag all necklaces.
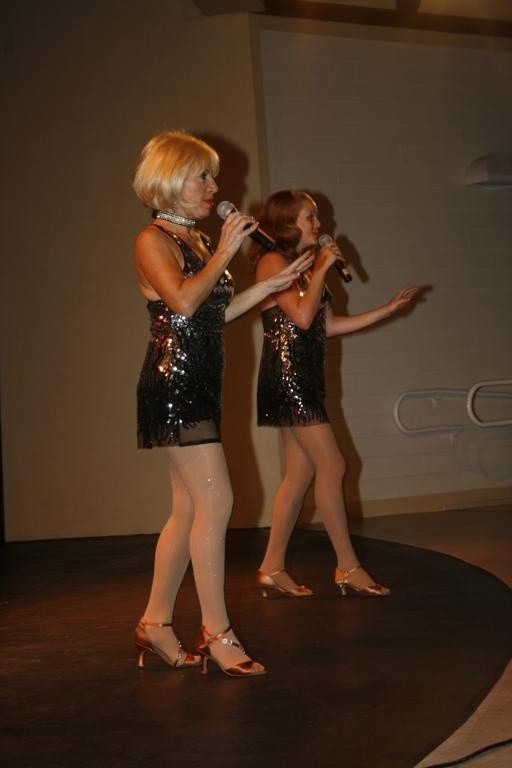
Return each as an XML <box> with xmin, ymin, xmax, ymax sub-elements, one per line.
<box><xmin>152</xmin><ymin>208</ymin><xmax>198</xmax><ymax>228</ymax></box>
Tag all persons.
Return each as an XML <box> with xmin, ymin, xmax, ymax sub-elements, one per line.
<box><xmin>130</xmin><ymin>128</ymin><xmax>316</xmax><ymax>681</ymax></box>
<box><xmin>250</xmin><ymin>188</ymin><xmax>416</xmax><ymax>600</ymax></box>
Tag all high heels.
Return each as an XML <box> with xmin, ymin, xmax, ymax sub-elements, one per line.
<box><xmin>199</xmin><ymin>623</ymin><xmax>266</xmax><ymax>676</ymax></box>
<box><xmin>258</xmin><ymin>568</ymin><xmax>316</xmax><ymax>599</ymax></box>
<box><xmin>132</xmin><ymin>619</ymin><xmax>202</xmax><ymax>667</ymax></box>
<box><xmin>336</xmin><ymin>567</ymin><xmax>390</xmax><ymax>597</ymax></box>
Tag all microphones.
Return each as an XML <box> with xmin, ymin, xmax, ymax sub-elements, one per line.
<box><xmin>318</xmin><ymin>233</ymin><xmax>353</xmax><ymax>283</ymax></box>
<box><xmin>216</xmin><ymin>200</ymin><xmax>277</xmax><ymax>252</ymax></box>
